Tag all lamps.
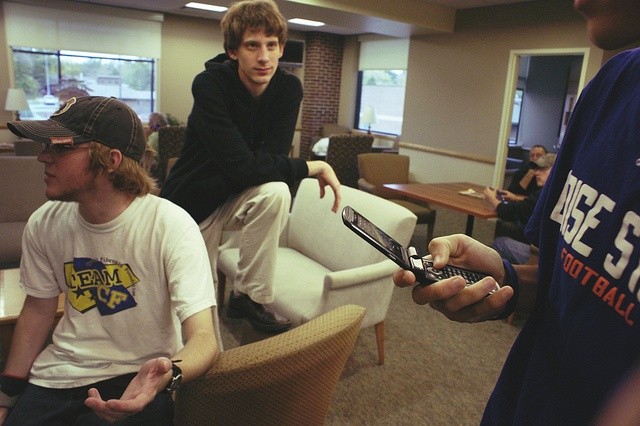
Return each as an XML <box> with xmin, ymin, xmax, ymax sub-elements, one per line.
<box><xmin>5</xmin><ymin>89</ymin><xmax>29</xmax><ymax>120</ymax></box>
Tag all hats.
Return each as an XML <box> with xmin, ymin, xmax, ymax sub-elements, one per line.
<box><xmin>528</xmin><ymin>153</ymin><xmax>558</xmax><ymax>168</ymax></box>
<box><xmin>7</xmin><ymin>96</ymin><xmax>145</xmax><ymax>162</ymax></box>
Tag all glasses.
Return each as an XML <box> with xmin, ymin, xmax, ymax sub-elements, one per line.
<box><xmin>41</xmin><ymin>141</ymin><xmax>90</xmax><ymax>154</ymax></box>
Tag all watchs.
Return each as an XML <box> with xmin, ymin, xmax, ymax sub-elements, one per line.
<box><xmin>165</xmin><ymin>360</ymin><xmax>183</xmax><ymax>394</ymax></box>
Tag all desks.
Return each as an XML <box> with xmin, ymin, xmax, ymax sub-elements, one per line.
<box><xmin>370</xmin><ymin>138</ymin><xmax>398</xmax><ymax>150</ymax></box>
<box><xmin>0</xmin><ymin>266</ymin><xmax>66</xmax><ymax>349</ymax></box>
<box><xmin>383</xmin><ymin>182</ymin><xmax>530</xmax><ymax>237</ymax></box>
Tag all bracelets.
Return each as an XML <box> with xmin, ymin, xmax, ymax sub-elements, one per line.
<box><xmin>0</xmin><ymin>375</ymin><xmax>27</xmax><ymax>410</ymax></box>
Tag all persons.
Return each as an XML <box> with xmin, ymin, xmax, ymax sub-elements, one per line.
<box><xmin>159</xmin><ymin>0</ymin><xmax>342</xmax><ymax>333</ymax></box>
<box><xmin>484</xmin><ymin>153</ymin><xmax>560</xmax><ymax>263</ymax></box>
<box><xmin>147</xmin><ymin>113</ymin><xmax>169</xmax><ymax>152</ymax></box>
<box><xmin>393</xmin><ymin>1</ymin><xmax>640</xmax><ymax>426</ymax></box>
<box><xmin>0</xmin><ymin>95</ymin><xmax>224</xmax><ymax>426</ymax></box>
<box><xmin>507</xmin><ymin>145</ymin><xmax>550</xmax><ymax>197</ymax></box>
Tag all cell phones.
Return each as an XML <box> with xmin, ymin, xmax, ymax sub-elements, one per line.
<box><xmin>341</xmin><ymin>204</ymin><xmax>502</xmax><ymax>301</ymax></box>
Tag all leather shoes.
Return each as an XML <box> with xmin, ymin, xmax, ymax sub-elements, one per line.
<box><xmin>227</xmin><ymin>290</ymin><xmax>291</xmax><ymax>333</ymax></box>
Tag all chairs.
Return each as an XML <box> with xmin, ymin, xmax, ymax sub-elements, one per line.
<box><xmin>175</xmin><ymin>303</ymin><xmax>366</xmax><ymax>425</ymax></box>
<box><xmin>159</xmin><ymin>126</ymin><xmax>187</xmax><ymax>179</ymax></box>
<box><xmin>357</xmin><ymin>153</ymin><xmax>436</xmax><ymax>250</ymax></box>
<box><xmin>326</xmin><ymin>134</ymin><xmax>374</xmax><ymax>185</ymax></box>
<box><xmin>507</xmin><ymin>243</ymin><xmax>539</xmax><ymax>324</ymax></box>
<box><xmin>15</xmin><ymin>139</ymin><xmax>42</xmax><ymax>156</ymax></box>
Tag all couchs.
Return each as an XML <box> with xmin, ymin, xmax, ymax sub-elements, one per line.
<box><xmin>1</xmin><ymin>156</ymin><xmax>50</xmax><ymax>266</ymax></box>
<box><xmin>222</xmin><ymin>178</ymin><xmax>418</xmax><ymax>364</ymax></box>
